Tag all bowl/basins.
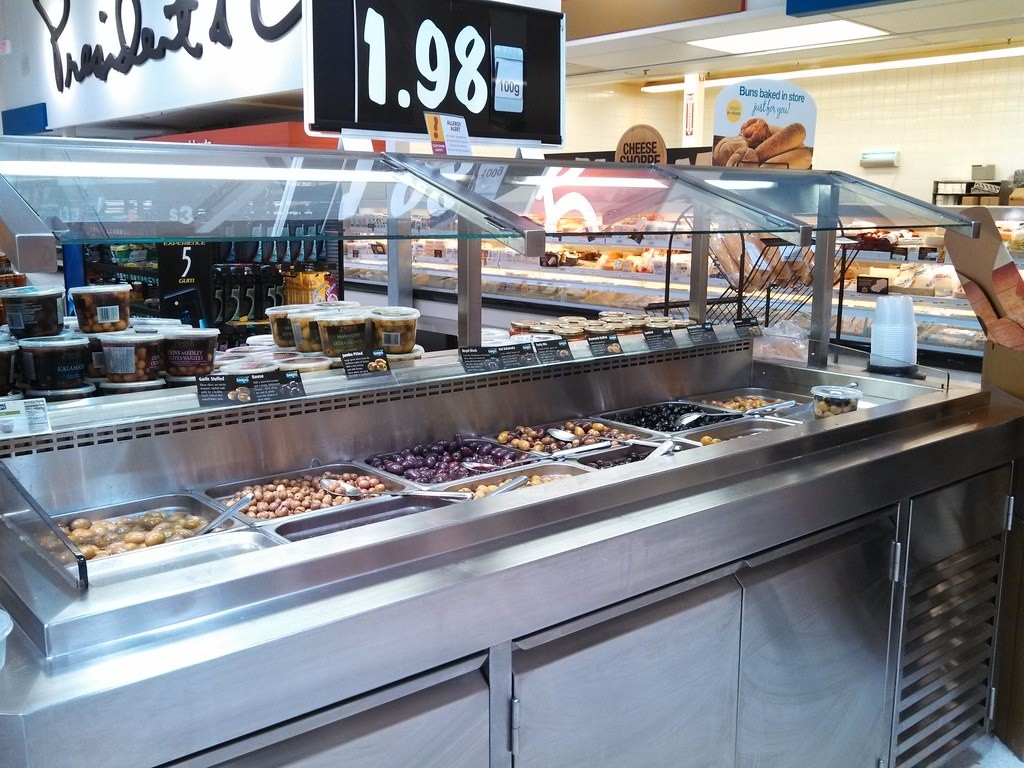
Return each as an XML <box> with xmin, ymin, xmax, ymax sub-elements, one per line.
<box><xmin>1</xmin><ymin>284</ymin><xmax>424</xmax><ymax>403</ymax></box>
<box><xmin>810</xmin><ymin>385</ymin><xmax>862</xmax><ymax>418</ymax></box>
<box><xmin>870</xmin><ymin>295</ymin><xmax>917</xmax><ymax>367</ymax></box>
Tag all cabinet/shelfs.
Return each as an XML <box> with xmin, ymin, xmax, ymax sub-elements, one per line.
<box><xmin>84</xmin><ymin>219</ymin><xmax>343</xmax><ymax>352</ymax></box>
<box><xmin>343</xmin><ymin>203</ymin><xmax>1024</xmax><ymax>374</ymax></box>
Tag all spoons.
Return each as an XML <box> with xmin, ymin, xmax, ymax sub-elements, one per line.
<box><xmin>320</xmin><ymin>478</ymin><xmax>474</xmax><ymax>503</ymax></box>
<box><xmin>548</xmin><ymin>427</ymin><xmax>682</xmax><ymax>451</ymax></box>
<box><xmin>461</xmin><ymin>452</ymin><xmax>565</xmax><ymax>472</ymax></box>
<box><xmin>674</xmin><ymin>411</ymin><xmax>763</xmax><ymax>428</ymax></box>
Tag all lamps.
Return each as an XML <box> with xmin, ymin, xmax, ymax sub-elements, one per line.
<box><xmin>642</xmin><ymin>41</ymin><xmax>1024</xmax><ymax>92</ymax></box>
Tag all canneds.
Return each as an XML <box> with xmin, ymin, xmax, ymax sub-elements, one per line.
<box><xmin>90</xmin><ymin>244</ymin><xmax>157</xmax><ymax>305</ymax></box>
<box><xmin>0</xmin><ymin>252</ymin><xmax>27</xmax><ymax>325</ymax></box>
<box><xmin>509</xmin><ymin>311</ymin><xmax>697</xmax><ymax>343</ymax></box>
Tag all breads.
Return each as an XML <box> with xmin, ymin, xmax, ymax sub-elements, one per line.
<box><xmin>712</xmin><ymin>117</ymin><xmax>812</xmax><ymax>170</ymax></box>
<box><xmin>601</xmin><ymin>248</ymin><xmax>680</xmax><ymax>275</ymax></box>
<box><xmin>710</xmin><ymin>220</ymin><xmax>841</xmax><ymax>291</ymax></box>
